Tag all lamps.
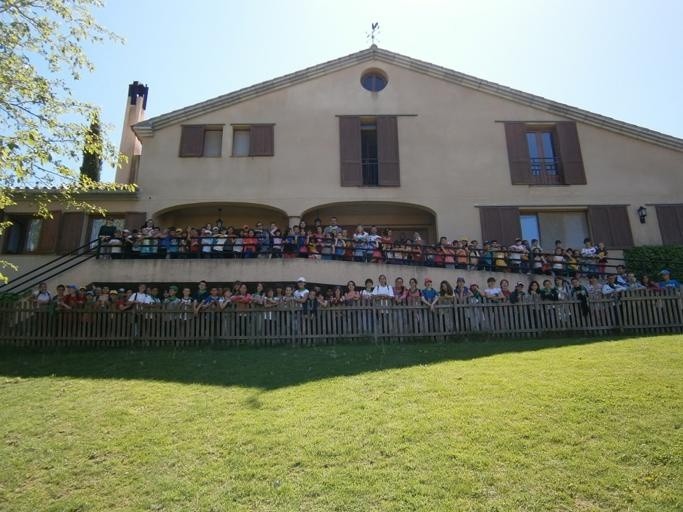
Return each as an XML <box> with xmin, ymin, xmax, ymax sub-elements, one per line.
<box><xmin>637</xmin><ymin>205</ymin><xmax>646</xmax><ymax>223</ymax></box>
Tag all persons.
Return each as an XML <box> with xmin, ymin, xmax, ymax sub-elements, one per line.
<box><xmin>33</xmin><ymin>264</ymin><xmax>678</xmax><ymax>340</ymax></box>
<box><xmin>98</xmin><ymin>217</ymin><xmax>608</xmax><ymax>279</ymax></box>
